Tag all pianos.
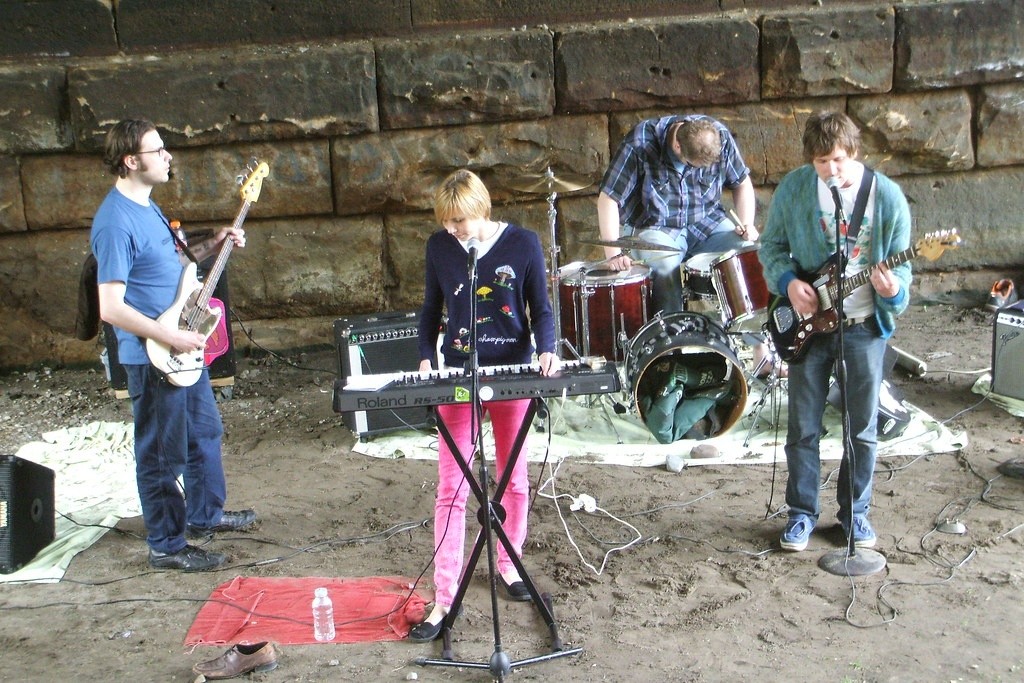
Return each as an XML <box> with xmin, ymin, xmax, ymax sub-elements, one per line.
<box><xmin>332</xmin><ymin>358</ymin><xmax>622</xmax><ymax>414</ymax></box>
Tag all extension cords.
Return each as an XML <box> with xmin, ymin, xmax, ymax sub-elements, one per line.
<box><xmin>891</xmin><ymin>346</ymin><xmax>927</xmax><ymax>377</ymax></box>
<box><xmin>579</xmin><ymin>492</ymin><xmax>596</xmax><ymax>513</ymax></box>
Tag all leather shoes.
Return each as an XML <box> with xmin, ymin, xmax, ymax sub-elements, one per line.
<box><xmin>193</xmin><ymin>642</ymin><xmax>279</xmax><ymax>679</ymax></box>
<box><xmin>186</xmin><ymin>510</ymin><xmax>256</xmax><ymax>536</ymax></box>
<box><xmin>147</xmin><ymin>545</ymin><xmax>225</xmax><ymax>570</ymax></box>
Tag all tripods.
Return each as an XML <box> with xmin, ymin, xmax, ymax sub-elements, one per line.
<box><xmin>741</xmin><ymin>336</ymin><xmax>828</xmax><ymax>448</ymax></box>
<box><xmin>415</xmin><ymin>266</ymin><xmax>583</xmax><ymax>683</ymax></box>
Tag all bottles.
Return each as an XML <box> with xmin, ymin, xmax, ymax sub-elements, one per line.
<box><xmin>312</xmin><ymin>588</ymin><xmax>335</xmax><ymax>642</ymax></box>
<box><xmin>170</xmin><ymin>220</ymin><xmax>188</xmax><ymax>255</ymax></box>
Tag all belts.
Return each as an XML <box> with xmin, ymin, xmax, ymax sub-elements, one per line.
<box><xmin>843</xmin><ymin>316</ymin><xmax>874</xmax><ymax>327</ymax></box>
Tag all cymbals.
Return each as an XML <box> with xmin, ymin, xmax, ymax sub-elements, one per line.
<box><xmin>576</xmin><ymin>235</ymin><xmax>682</xmax><ymax>256</ymax></box>
<box><xmin>508</xmin><ymin>171</ymin><xmax>593</xmax><ymax>197</ymax></box>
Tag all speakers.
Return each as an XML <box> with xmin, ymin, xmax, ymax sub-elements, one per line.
<box><xmin>99</xmin><ymin>252</ymin><xmax>236</xmax><ymax>390</ymax></box>
<box><xmin>332</xmin><ymin>308</ymin><xmax>442</xmax><ymax>439</ymax></box>
<box><xmin>990</xmin><ymin>299</ymin><xmax>1024</xmax><ymax>401</ymax></box>
<box><xmin>0</xmin><ymin>455</ymin><xmax>56</xmax><ymax>575</ymax></box>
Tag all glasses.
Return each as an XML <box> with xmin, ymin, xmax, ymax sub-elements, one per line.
<box><xmin>136</xmin><ymin>147</ymin><xmax>165</xmax><ymax>156</ymax></box>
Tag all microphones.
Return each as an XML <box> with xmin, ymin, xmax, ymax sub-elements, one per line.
<box><xmin>467</xmin><ymin>237</ymin><xmax>478</xmax><ymax>279</ymax></box>
<box><xmin>826</xmin><ymin>175</ymin><xmax>848</xmax><ymax>225</ymax></box>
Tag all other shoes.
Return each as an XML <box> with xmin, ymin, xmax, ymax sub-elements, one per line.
<box><xmin>985</xmin><ymin>279</ymin><xmax>1019</xmax><ymax>310</ymax></box>
<box><xmin>408</xmin><ymin>602</ymin><xmax>463</xmax><ymax>641</ymax></box>
<box><xmin>499</xmin><ymin>573</ymin><xmax>532</xmax><ymax>600</ymax></box>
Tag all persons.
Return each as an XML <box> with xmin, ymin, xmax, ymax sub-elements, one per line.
<box><xmin>409</xmin><ymin>168</ymin><xmax>564</xmax><ymax>644</ymax></box>
<box><xmin>91</xmin><ymin>120</ymin><xmax>255</xmax><ymax>571</ymax></box>
<box><xmin>759</xmin><ymin>109</ymin><xmax>913</xmax><ymax>552</ymax></box>
<box><xmin>597</xmin><ymin>113</ymin><xmax>793</xmax><ymax>383</ymax></box>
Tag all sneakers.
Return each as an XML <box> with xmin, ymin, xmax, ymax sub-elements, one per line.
<box><xmin>780</xmin><ymin>513</ymin><xmax>816</xmax><ymax>551</ymax></box>
<box><xmin>842</xmin><ymin>513</ymin><xmax>876</xmax><ymax>547</ymax></box>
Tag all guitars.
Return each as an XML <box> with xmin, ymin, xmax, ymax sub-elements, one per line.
<box><xmin>766</xmin><ymin>227</ymin><xmax>962</xmax><ymax>363</ymax></box>
<box><xmin>144</xmin><ymin>158</ymin><xmax>270</xmax><ymax>388</ymax></box>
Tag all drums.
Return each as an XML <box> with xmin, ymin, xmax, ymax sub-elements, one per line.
<box><xmin>555</xmin><ymin>257</ymin><xmax>655</xmax><ymax>362</ymax></box>
<box><xmin>707</xmin><ymin>239</ymin><xmax>773</xmax><ymax>340</ymax></box>
<box><xmin>683</xmin><ymin>252</ymin><xmax>731</xmax><ymax>318</ymax></box>
